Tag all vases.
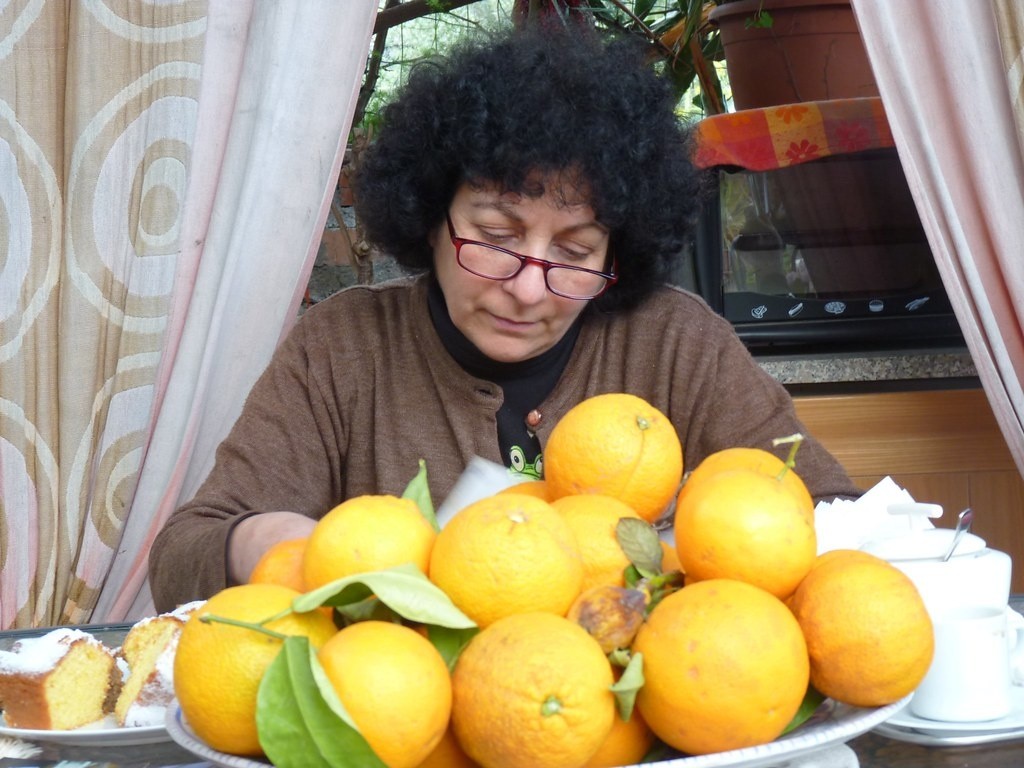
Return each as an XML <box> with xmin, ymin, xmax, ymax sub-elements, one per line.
<box><xmin>710</xmin><ymin>0</ymin><xmax>881</xmax><ymax>113</ymax></box>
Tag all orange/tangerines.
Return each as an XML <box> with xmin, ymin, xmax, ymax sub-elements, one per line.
<box><xmin>175</xmin><ymin>391</ymin><xmax>934</xmax><ymax>768</ymax></box>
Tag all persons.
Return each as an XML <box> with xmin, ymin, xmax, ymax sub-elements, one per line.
<box><xmin>147</xmin><ymin>22</ymin><xmax>866</xmax><ymax>616</ymax></box>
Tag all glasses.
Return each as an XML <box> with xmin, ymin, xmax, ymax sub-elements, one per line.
<box><xmin>443</xmin><ymin>212</ymin><xmax>619</xmax><ymax>300</ymax></box>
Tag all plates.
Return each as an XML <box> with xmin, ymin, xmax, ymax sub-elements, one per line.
<box><xmin>0</xmin><ymin>710</ymin><xmax>177</xmax><ymax>747</ymax></box>
<box><xmin>162</xmin><ymin>597</ymin><xmax>922</xmax><ymax>768</ymax></box>
<box><xmin>866</xmin><ymin>716</ymin><xmax>1024</xmax><ymax>745</ymax></box>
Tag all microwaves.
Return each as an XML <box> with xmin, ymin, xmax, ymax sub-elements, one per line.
<box><xmin>690</xmin><ymin>98</ymin><xmax>965</xmax><ymax>353</ymax></box>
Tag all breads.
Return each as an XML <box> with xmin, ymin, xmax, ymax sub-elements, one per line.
<box><xmin>115</xmin><ymin>599</ymin><xmax>213</xmax><ymax>724</ymax></box>
<box><xmin>0</xmin><ymin>627</ymin><xmax>124</xmax><ymax>733</ymax></box>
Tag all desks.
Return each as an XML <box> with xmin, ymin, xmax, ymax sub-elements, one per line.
<box><xmin>0</xmin><ymin>592</ymin><xmax>1023</xmax><ymax>768</ymax></box>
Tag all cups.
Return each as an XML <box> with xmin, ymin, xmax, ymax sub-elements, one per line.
<box><xmin>909</xmin><ymin>605</ymin><xmax>1024</xmax><ymax>723</ymax></box>
<box><xmin>871</xmin><ymin>528</ymin><xmax>1012</xmax><ymax>623</ymax></box>
